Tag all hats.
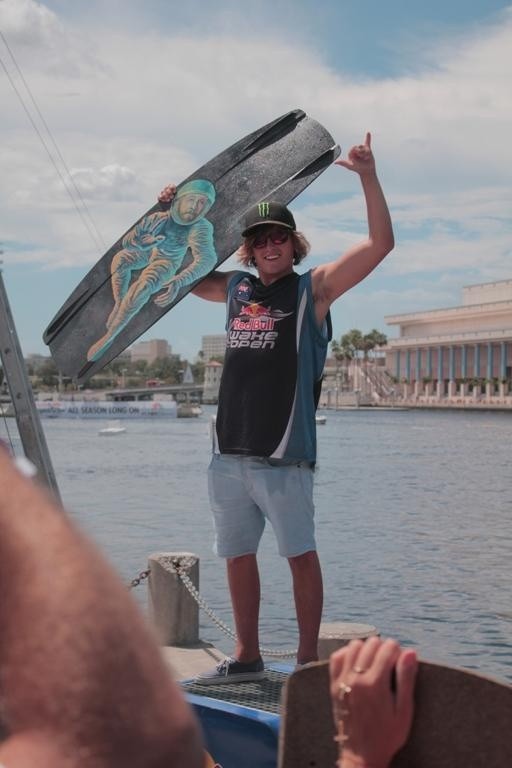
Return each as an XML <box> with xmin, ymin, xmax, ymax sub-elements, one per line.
<box><xmin>241</xmin><ymin>201</ymin><xmax>297</xmax><ymax>237</ymax></box>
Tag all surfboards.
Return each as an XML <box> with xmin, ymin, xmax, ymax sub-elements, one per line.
<box><xmin>42</xmin><ymin>109</ymin><xmax>341</xmax><ymax>386</ymax></box>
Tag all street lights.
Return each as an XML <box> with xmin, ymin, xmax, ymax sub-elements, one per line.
<box><xmin>121</xmin><ymin>368</ymin><xmax>128</xmax><ymax>389</ymax></box>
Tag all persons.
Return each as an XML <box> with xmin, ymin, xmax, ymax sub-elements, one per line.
<box><xmin>2</xmin><ymin>441</ymin><xmax>214</xmax><ymax>768</ymax></box>
<box><xmin>327</xmin><ymin>632</ymin><xmax>418</xmax><ymax>767</ymax></box>
<box><xmin>158</xmin><ymin>129</ymin><xmax>395</xmax><ymax>686</ymax></box>
<box><xmin>87</xmin><ymin>178</ymin><xmax>218</xmax><ymax>363</ymax></box>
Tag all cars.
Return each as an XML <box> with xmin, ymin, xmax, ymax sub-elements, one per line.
<box><xmin>144</xmin><ymin>375</ymin><xmax>161</xmax><ymax>387</ymax></box>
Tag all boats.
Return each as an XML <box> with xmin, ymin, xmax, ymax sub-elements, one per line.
<box><xmin>95</xmin><ymin>417</ymin><xmax>128</xmax><ymax>435</ymax></box>
<box><xmin>315</xmin><ymin>413</ymin><xmax>327</xmax><ymax>425</ymax></box>
<box><xmin>175</xmin><ymin>397</ymin><xmax>202</xmax><ymax>417</ymax></box>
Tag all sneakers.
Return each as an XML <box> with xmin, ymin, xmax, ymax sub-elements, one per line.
<box><xmin>193</xmin><ymin>654</ymin><xmax>266</xmax><ymax>686</ymax></box>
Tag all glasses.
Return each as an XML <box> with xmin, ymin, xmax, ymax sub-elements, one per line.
<box><xmin>252</xmin><ymin>229</ymin><xmax>289</xmax><ymax>248</ymax></box>
